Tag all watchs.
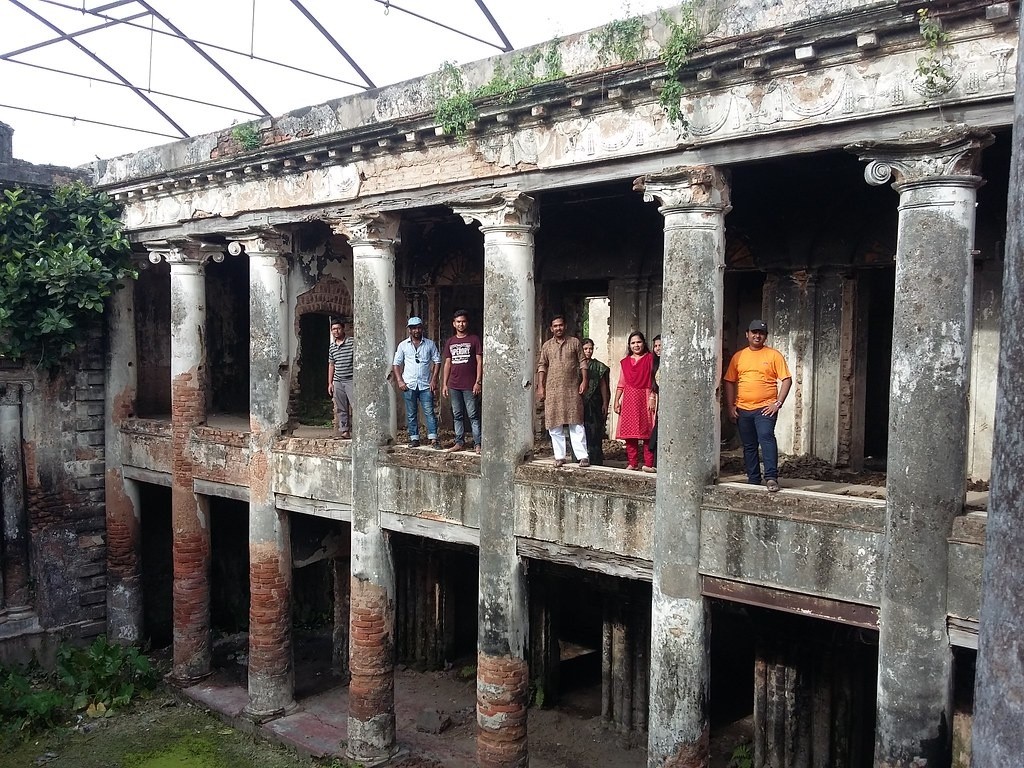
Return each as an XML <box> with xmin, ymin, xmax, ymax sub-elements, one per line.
<box><xmin>476</xmin><ymin>379</ymin><xmax>483</xmax><ymax>385</ymax></box>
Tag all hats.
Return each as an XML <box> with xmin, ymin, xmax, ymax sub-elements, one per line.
<box><xmin>405</xmin><ymin>317</ymin><xmax>423</xmax><ymax>327</ymax></box>
<box><xmin>749</xmin><ymin>319</ymin><xmax>767</xmax><ymax>332</ymax></box>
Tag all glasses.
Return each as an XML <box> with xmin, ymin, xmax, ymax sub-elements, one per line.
<box><xmin>414</xmin><ymin>353</ymin><xmax>420</xmax><ymax>363</ymax></box>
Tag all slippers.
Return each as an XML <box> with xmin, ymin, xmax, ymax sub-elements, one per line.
<box><xmin>334</xmin><ymin>435</ymin><xmax>351</xmax><ymax>440</ymax></box>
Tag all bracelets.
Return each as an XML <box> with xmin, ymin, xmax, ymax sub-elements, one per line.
<box><xmin>774</xmin><ymin>400</ymin><xmax>783</xmax><ymax>408</ymax></box>
<box><xmin>649</xmin><ymin>397</ymin><xmax>656</xmax><ymax>401</ymax></box>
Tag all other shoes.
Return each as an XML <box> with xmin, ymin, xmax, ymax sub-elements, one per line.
<box><xmin>642</xmin><ymin>465</ymin><xmax>657</xmax><ymax>473</ymax></box>
<box><xmin>432</xmin><ymin>442</ymin><xmax>442</xmax><ymax>450</ymax></box>
<box><xmin>447</xmin><ymin>442</ymin><xmax>463</xmax><ymax>453</ymax></box>
<box><xmin>626</xmin><ymin>465</ymin><xmax>639</xmax><ymax>470</ymax></box>
<box><xmin>553</xmin><ymin>459</ymin><xmax>566</xmax><ymax>467</ymax></box>
<box><xmin>408</xmin><ymin>439</ymin><xmax>421</xmax><ymax>448</ymax></box>
<box><xmin>474</xmin><ymin>445</ymin><xmax>481</xmax><ymax>454</ymax></box>
<box><xmin>578</xmin><ymin>458</ymin><xmax>590</xmax><ymax>467</ymax></box>
<box><xmin>767</xmin><ymin>480</ymin><xmax>779</xmax><ymax>492</ymax></box>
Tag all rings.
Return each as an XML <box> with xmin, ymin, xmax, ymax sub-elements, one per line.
<box><xmin>443</xmin><ymin>393</ymin><xmax>446</xmax><ymax>394</ymax></box>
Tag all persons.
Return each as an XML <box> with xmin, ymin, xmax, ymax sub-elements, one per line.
<box><xmin>442</xmin><ymin>310</ymin><xmax>484</xmax><ymax>454</ymax></box>
<box><xmin>724</xmin><ymin>320</ymin><xmax>792</xmax><ymax>492</ymax></box>
<box><xmin>642</xmin><ymin>333</ymin><xmax>662</xmax><ymax>472</ymax></box>
<box><xmin>538</xmin><ymin>314</ymin><xmax>590</xmax><ymax>468</ymax></box>
<box><xmin>327</xmin><ymin>319</ymin><xmax>355</xmax><ymax>440</ymax></box>
<box><xmin>577</xmin><ymin>337</ymin><xmax>611</xmax><ymax>465</ymax></box>
<box><xmin>614</xmin><ymin>331</ymin><xmax>659</xmax><ymax>472</ymax></box>
<box><xmin>392</xmin><ymin>316</ymin><xmax>442</xmax><ymax>449</ymax></box>
